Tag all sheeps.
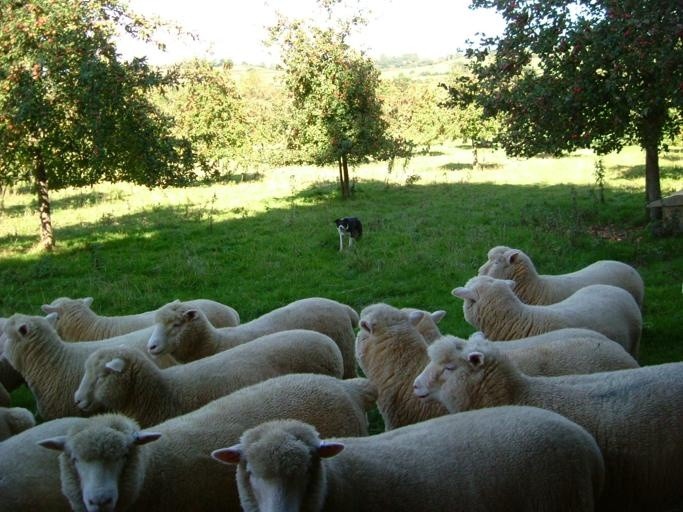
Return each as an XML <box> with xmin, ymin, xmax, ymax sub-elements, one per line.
<box><xmin>149</xmin><ymin>298</ymin><xmax>360</xmax><ymax>381</ymax></box>
<box><xmin>402</xmin><ymin>307</ymin><xmax>610</xmax><ymax>349</ymax></box>
<box><xmin>41</xmin><ymin>296</ymin><xmax>243</xmax><ymax>342</ymax></box>
<box><xmin>211</xmin><ymin>404</ymin><xmax>606</xmax><ymax>511</ymax></box>
<box><xmin>0</xmin><ymin>413</ymin><xmax>87</xmax><ymax>512</ymax></box>
<box><xmin>0</xmin><ymin>312</ymin><xmax>179</xmax><ymax>421</ymax></box>
<box><xmin>452</xmin><ymin>276</ymin><xmax>641</xmax><ymax>353</ymax></box>
<box><xmin>413</xmin><ymin>331</ymin><xmax>683</xmax><ymax>512</ymax></box>
<box><xmin>477</xmin><ymin>246</ymin><xmax>644</xmax><ymax>309</ymax></box>
<box><xmin>36</xmin><ymin>372</ymin><xmax>379</xmax><ymax>512</ymax></box>
<box><xmin>353</xmin><ymin>304</ymin><xmax>641</xmax><ymax>432</ymax></box>
<box><xmin>74</xmin><ymin>328</ymin><xmax>344</xmax><ymax>427</ymax></box>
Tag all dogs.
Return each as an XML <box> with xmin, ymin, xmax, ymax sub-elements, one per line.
<box><xmin>334</xmin><ymin>217</ymin><xmax>363</xmax><ymax>253</ymax></box>
